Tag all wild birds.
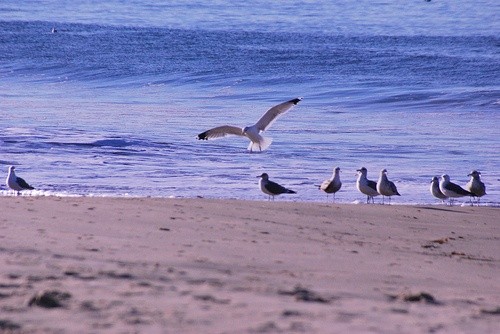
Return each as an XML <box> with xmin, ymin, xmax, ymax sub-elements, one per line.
<box><xmin>321</xmin><ymin>167</ymin><xmax>342</xmax><ymax>198</ymax></box>
<box><xmin>356</xmin><ymin>167</ymin><xmax>402</xmax><ymax>206</ymax></box>
<box><xmin>194</xmin><ymin>96</ymin><xmax>304</xmax><ymax>152</ymax></box>
<box><xmin>430</xmin><ymin>170</ymin><xmax>487</xmax><ymax>207</ymax></box>
<box><xmin>6</xmin><ymin>165</ymin><xmax>35</xmax><ymax>197</ymax></box>
<box><xmin>256</xmin><ymin>173</ymin><xmax>297</xmax><ymax>200</ymax></box>
